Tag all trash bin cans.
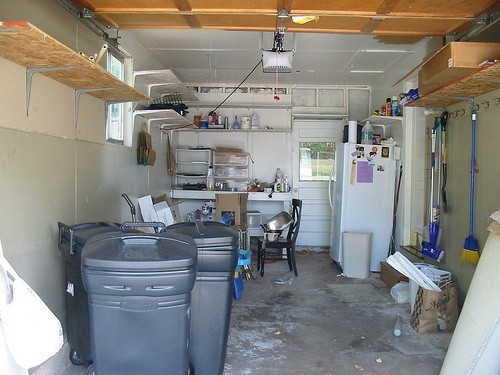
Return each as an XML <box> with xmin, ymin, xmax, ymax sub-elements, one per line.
<box><xmin>161</xmin><ymin>220</ymin><xmax>238</xmax><ymax>375</ymax></box>
<box><xmin>57</xmin><ymin>221</ymin><xmax>148</xmax><ymax>364</ymax></box>
<box><xmin>342</xmin><ymin>231</ymin><xmax>372</xmax><ymax>280</ymax></box>
<box><xmin>80</xmin><ymin>222</ymin><xmax>197</xmax><ymax>375</ymax></box>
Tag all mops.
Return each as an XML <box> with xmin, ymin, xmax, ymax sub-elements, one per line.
<box><xmin>420</xmin><ymin>122</ymin><xmax>445</xmax><ymax>263</ymax></box>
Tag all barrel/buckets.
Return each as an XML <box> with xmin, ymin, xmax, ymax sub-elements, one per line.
<box><xmin>240</xmin><ymin>116</ymin><xmax>250</xmax><ymax>129</ymax></box>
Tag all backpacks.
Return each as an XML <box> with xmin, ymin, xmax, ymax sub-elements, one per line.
<box><xmin>137</xmin><ymin>123</ymin><xmax>156</xmax><ymax>166</ymax></box>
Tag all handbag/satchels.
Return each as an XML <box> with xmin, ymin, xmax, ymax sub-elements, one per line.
<box><xmin>410</xmin><ymin>281</ymin><xmax>459</xmax><ymax>335</ymax></box>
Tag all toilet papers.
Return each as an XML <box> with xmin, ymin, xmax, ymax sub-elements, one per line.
<box><xmin>348</xmin><ymin>120</ymin><xmax>357</xmax><ymax>144</ymax></box>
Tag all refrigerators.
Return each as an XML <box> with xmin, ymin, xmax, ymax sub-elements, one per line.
<box><xmin>328</xmin><ymin>143</ymin><xmax>396</xmax><ymax>273</ymax></box>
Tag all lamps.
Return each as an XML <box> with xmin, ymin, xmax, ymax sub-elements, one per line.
<box><xmin>278</xmin><ymin>9</ymin><xmax>290</xmax><ymax>18</ymax></box>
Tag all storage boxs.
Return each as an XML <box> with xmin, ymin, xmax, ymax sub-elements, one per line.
<box><xmin>138</xmin><ymin>194</ymin><xmax>184</xmax><ymax>234</ymax></box>
<box><xmin>418</xmin><ymin>42</ymin><xmax>500</xmax><ymax>96</ymax></box>
<box><xmin>380</xmin><ymin>262</ymin><xmax>409</xmax><ymax>289</ymax></box>
<box><xmin>247</xmin><ymin>210</ymin><xmax>262</xmax><ymax>230</ymax></box>
<box><xmin>215</xmin><ymin>193</ymin><xmax>249</xmax><ymax>225</ymax></box>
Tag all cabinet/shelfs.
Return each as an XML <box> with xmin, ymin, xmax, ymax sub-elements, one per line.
<box><xmin>134</xmin><ymin>70</ymin><xmax>200</xmax><ymax>130</ymax></box>
<box><xmin>212</xmin><ymin>150</ymin><xmax>249</xmax><ymax>192</ymax></box>
<box><xmin>175</xmin><ymin>149</ymin><xmax>213</xmax><ymax>189</ymax></box>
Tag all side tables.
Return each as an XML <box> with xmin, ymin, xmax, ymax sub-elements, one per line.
<box><xmin>175</xmin><ymin>104</ymin><xmax>293</xmax><ymax>132</ymax></box>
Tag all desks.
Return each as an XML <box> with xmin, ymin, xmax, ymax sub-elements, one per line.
<box><xmin>247</xmin><ymin>226</ymin><xmax>263</xmax><ymax>250</ymax></box>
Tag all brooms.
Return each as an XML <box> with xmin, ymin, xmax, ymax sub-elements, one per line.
<box><xmin>459</xmin><ymin>109</ymin><xmax>480</xmax><ymax>265</ymax></box>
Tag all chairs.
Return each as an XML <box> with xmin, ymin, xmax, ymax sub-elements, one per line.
<box><xmin>256</xmin><ymin>199</ymin><xmax>302</xmax><ymax>277</ymax></box>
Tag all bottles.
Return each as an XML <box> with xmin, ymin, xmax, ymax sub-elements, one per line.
<box><xmin>234</xmin><ymin>115</ymin><xmax>239</xmax><ymax>129</ymax></box>
<box><xmin>251</xmin><ymin>113</ymin><xmax>259</xmax><ymax>129</ymax></box>
<box><xmin>386</xmin><ymin>97</ymin><xmax>392</xmax><ymax>116</ymax></box>
<box><xmin>224</xmin><ymin>116</ymin><xmax>229</xmax><ymax>129</ymax></box>
<box><xmin>206</xmin><ymin>166</ymin><xmax>215</xmax><ymax>190</ymax></box>
<box><xmin>199</xmin><ymin>114</ymin><xmax>208</xmax><ymax>128</ymax></box>
<box><xmin>392</xmin><ymin>96</ymin><xmax>398</xmax><ymax>116</ymax></box>
<box><xmin>254</xmin><ymin>179</ymin><xmax>260</xmax><ymax>188</ymax></box>
<box><xmin>208</xmin><ymin>111</ymin><xmax>222</xmax><ymax>124</ymax></box>
<box><xmin>273</xmin><ymin>168</ymin><xmax>289</xmax><ymax>192</ymax></box>
<box><xmin>202</xmin><ymin>203</ymin><xmax>210</xmax><ymax>215</ymax></box>
<box><xmin>361</xmin><ymin>121</ymin><xmax>374</xmax><ymax>145</ymax></box>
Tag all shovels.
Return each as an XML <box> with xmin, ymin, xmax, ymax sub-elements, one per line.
<box><xmin>441</xmin><ymin>111</ymin><xmax>449</xmax><ymax>213</ymax></box>
